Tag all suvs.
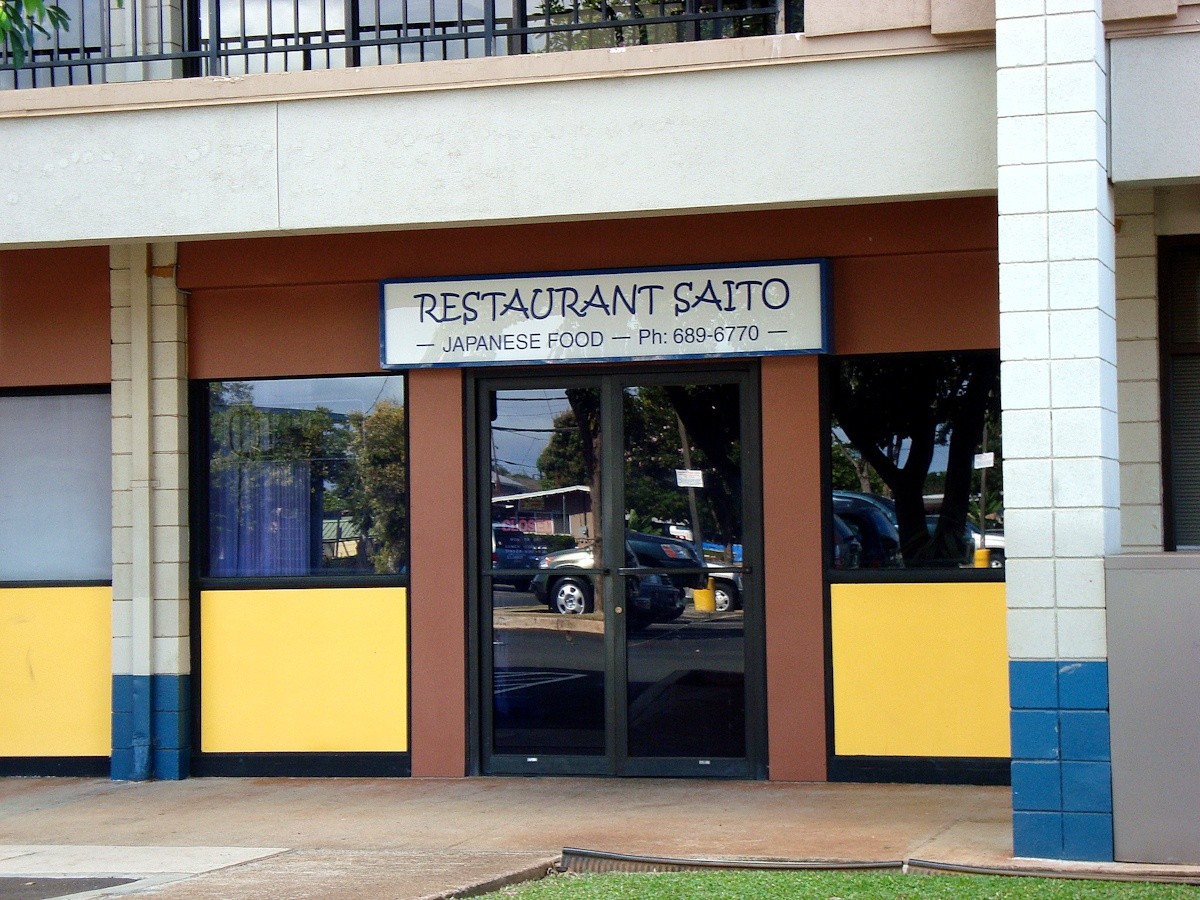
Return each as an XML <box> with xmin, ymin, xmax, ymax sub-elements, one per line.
<box><xmin>832</xmin><ymin>491</ymin><xmax>1006</xmax><ymax>574</ymax></box>
<box><xmin>492</xmin><ymin>525</ymin><xmax>532</xmax><ymax>591</ymax></box>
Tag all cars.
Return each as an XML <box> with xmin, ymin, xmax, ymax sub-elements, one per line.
<box><xmin>535</xmin><ymin>530</ymin><xmax>747</xmax><ymax>624</ymax></box>
